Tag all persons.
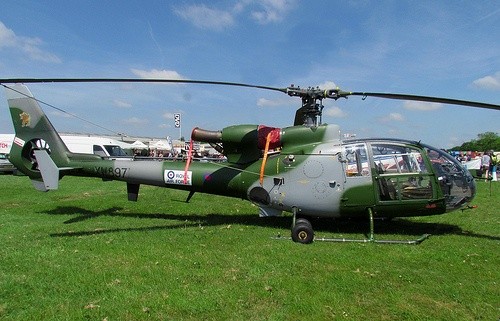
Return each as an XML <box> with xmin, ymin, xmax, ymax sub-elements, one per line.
<box><xmin>132</xmin><ymin>146</ymin><xmax>226</xmax><ymax>165</ymax></box>
<box><xmin>425</xmin><ymin>149</ymin><xmax>500</xmax><ymax>182</ymax></box>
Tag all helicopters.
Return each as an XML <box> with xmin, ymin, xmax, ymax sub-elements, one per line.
<box><xmin>1</xmin><ymin>78</ymin><xmax>500</xmax><ymax>247</ymax></box>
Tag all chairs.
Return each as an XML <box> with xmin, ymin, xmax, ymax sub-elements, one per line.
<box><xmin>378</xmin><ymin>162</ymin><xmax>413</xmax><ymax>200</ymax></box>
<box><xmin>373</xmin><ymin>163</ymin><xmax>386</xmax><ymax>198</ymax></box>
